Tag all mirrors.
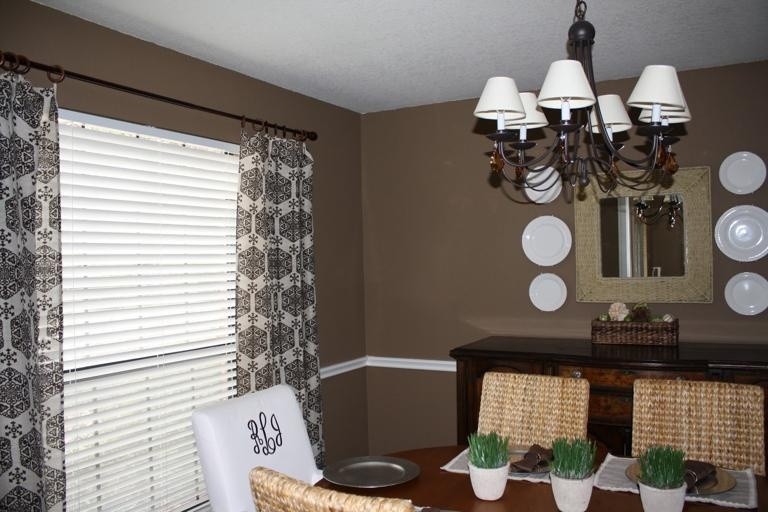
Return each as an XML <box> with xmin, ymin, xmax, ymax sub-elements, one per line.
<box><xmin>573</xmin><ymin>164</ymin><xmax>713</xmax><ymax>303</ymax></box>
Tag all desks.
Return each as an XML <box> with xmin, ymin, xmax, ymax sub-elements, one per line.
<box><xmin>448</xmin><ymin>335</ymin><xmax>768</xmax><ymax>456</ymax></box>
<box><xmin>314</xmin><ymin>445</ymin><xmax>768</xmax><ymax>512</ymax></box>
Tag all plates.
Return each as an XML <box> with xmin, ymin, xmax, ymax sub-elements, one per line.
<box><xmin>525</xmin><ymin>165</ymin><xmax>563</xmax><ymax>205</ymax></box>
<box><xmin>724</xmin><ymin>271</ymin><xmax>768</xmax><ymax>316</ymax></box>
<box><xmin>625</xmin><ymin>459</ymin><xmax>738</xmax><ymax>497</ymax></box>
<box><xmin>719</xmin><ymin>151</ymin><xmax>767</xmax><ymax>196</ymax></box>
<box><xmin>323</xmin><ymin>455</ymin><xmax>422</xmax><ymax>489</ymax></box>
<box><xmin>528</xmin><ymin>273</ymin><xmax>569</xmax><ymax>312</ymax></box>
<box><xmin>502</xmin><ymin>454</ymin><xmax>556</xmax><ymax>477</ymax></box>
<box><xmin>714</xmin><ymin>205</ymin><xmax>768</xmax><ymax>263</ymax></box>
<box><xmin>521</xmin><ymin>216</ymin><xmax>573</xmax><ymax>267</ymax></box>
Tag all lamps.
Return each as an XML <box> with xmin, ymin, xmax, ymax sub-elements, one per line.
<box><xmin>632</xmin><ymin>194</ymin><xmax>683</xmax><ymax>228</ymax></box>
<box><xmin>472</xmin><ymin>1</ymin><xmax>692</xmax><ymax>191</ymax></box>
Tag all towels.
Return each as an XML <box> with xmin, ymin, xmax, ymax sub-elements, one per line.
<box><xmin>510</xmin><ymin>444</ymin><xmax>552</xmax><ymax>472</ymax></box>
<box><xmin>684</xmin><ymin>459</ymin><xmax>716</xmax><ymax>491</ymax></box>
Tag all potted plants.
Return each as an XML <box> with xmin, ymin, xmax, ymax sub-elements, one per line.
<box><xmin>637</xmin><ymin>446</ymin><xmax>687</xmax><ymax>512</ymax></box>
<box><xmin>466</xmin><ymin>429</ymin><xmax>511</xmax><ymax>501</ymax></box>
<box><xmin>549</xmin><ymin>436</ymin><xmax>597</xmax><ymax>512</ymax></box>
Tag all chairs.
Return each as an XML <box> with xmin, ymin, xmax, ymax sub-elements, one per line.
<box><xmin>476</xmin><ymin>371</ymin><xmax>591</xmax><ymax>449</ymax></box>
<box><xmin>191</xmin><ymin>382</ymin><xmax>324</xmax><ymax>512</ymax></box>
<box><xmin>632</xmin><ymin>379</ymin><xmax>765</xmax><ymax>477</ymax></box>
<box><xmin>249</xmin><ymin>466</ymin><xmax>413</xmax><ymax>512</ymax></box>
